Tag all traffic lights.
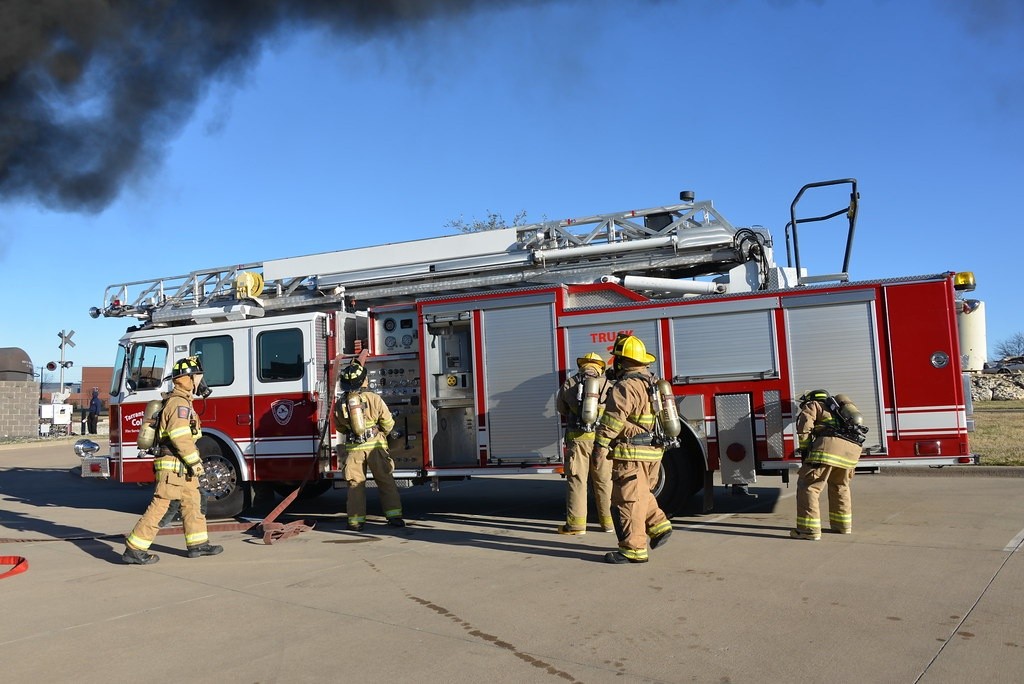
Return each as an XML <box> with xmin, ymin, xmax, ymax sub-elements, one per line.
<box><xmin>47</xmin><ymin>361</ymin><xmax>57</xmax><ymax>372</ymax></box>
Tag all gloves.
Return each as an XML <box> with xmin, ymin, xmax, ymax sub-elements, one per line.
<box><xmin>592</xmin><ymin>442</ymin><xmax>609</xmax><ymax>470</ymax></box>
<box><xmin>801</xmin><ymin>452</ymin><xmax>809</xmax><ymax>466</ymax></box>
<box><xmin>191</xmin><ymin>460</ymin><xmax>204</xmax><ymax>477</ymax></box>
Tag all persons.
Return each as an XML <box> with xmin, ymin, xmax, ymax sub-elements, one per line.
<box><xmin>591</xmin><ymin>335</ymin><xmax>673</xmax><ymax>564</ymax></box>
<box><xmin>122</xmin><ymin>356</ymin><xmax>223</xmax><ymax>565</ymax></box>
<box><xmin>334</xmin><ymin>359</ymin><xmax>405</xmax><ymax>530</ymax></box>
<box><xmin>789</xmin><ymin>389</ymin><xmax>863</xmax><ymax>540</ymax></box>
<box><xmin>557</xmin><ymin>352</ymin><xmax>615</xmax><ymax>534</ymax></box>
<box><xmin>87</xmin><ymin>391</ymin><xmax>100</xmax><ymax>435</ymax></box>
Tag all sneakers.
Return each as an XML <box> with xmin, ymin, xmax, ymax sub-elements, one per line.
<box><xmin>558</xmin><ymin>525</ymin><xmax>586</xmax><ymax>535</ymax></box>
<box><xmin>650</xmin><ymin>529</ymin><xmax>673</xmax><ymax>549</ymax></box>
<box><xmin>188</xmin><ymin>544</ymin><xmax>223</xmax><ymax>557</ymax></box>
<box><xmin>604</xmin><ymin>527</ymin><xmax>614</xmax><ymax>532</ymax></box>
<box><xmin>604</xmin><ymin>552</ymin><xmax>649</xmax><ymax>563</ymax></box>
<box><xmin>121</xmin><ymin>548</ymin><xmax>159</xmax><ymax>563</ymax></box>
<box><xmin>832</xmin><ymin>526</ymin><xmax>852</xmax><ymax>534</ymax></box>
<box><xmin>388</xmin><ymin>518</ymin><xmax>405</xmax><ymax>527</ymax></box>
<box><xmin>348</xmin><ymin>523</ymin><xmax>363</xmax><ymax>530</ymax></box>
<box><xmin>790</xmin><ymin>529</ymin><xmax>822</xmax><ymax>540</ymax></box>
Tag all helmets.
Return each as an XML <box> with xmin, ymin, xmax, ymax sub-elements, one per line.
<box><xmin>577</xmin><ymin>352</ymin><xmax>606</xmax><ymax>374</ymax></box>
<box><xmin>610</xmin><ymin>336</ymin><xmax>656</xmax><ymax>363</ymax></box>
<box><xmin>800</xmin><ymin>390</ymin><xmax>830</xmax><ymax>409</ymax></box>
<box><xmin>164</xmin><ymin>358</ymin><xmax>206</xmax><ymax>381</ymax></box>
<box><xmin>340</xmin><ymin>363</ymin><xmax>367</xmax><ymax>390</ymax></box>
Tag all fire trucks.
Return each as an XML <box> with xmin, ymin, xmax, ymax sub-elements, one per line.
<box><xmin>74</xmin><ymin>177</ymin><xmax>988</xmax><ymax>519</ymax></box>
